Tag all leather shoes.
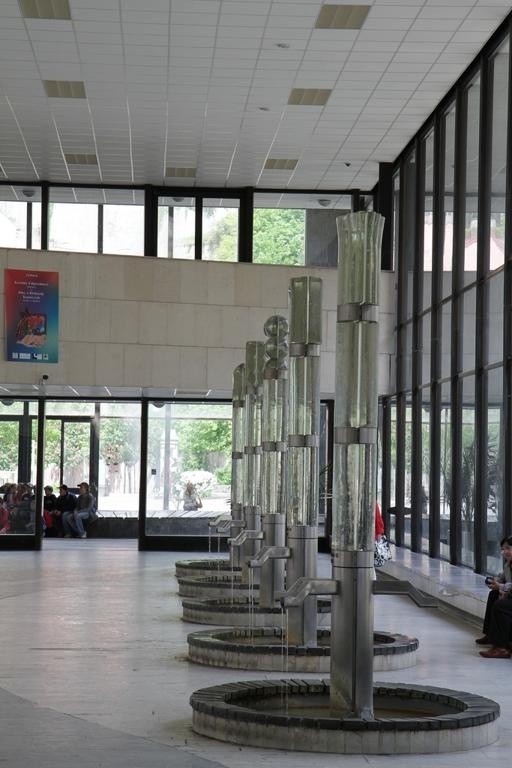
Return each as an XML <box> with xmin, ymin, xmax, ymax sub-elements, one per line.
<box><xmin>476</xmin><ymin>634</ymin><xmax>493</xmax><ymax>645</ymax></box>
<box><xmin>479</xmin><ymin>646</ymin><xmax>511</xmax><ymax>658</ymax></box>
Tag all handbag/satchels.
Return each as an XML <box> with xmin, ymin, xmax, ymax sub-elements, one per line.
<box><xmin>374</xmin><ymin>534</ymin><xmax>392</xmax><ymax>566</ymax></box>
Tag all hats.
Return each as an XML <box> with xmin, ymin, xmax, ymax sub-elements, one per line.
<box><xmin>58</xmin><ymin>485</ymin><xmax>67</xmax><ymax>490</ymax></box>
<box><xmin>77</xmin><ymin>482</ymin><xmax>88</xmax><ymax>487</ymax></box>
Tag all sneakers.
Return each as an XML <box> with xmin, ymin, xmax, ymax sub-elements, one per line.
<box><xmin>64</xmin><ymin>531</ymin><xmax>87</xmax><ymax>538</ymax></box>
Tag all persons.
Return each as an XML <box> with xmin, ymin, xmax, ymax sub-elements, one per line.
<box><xmin>182</xmin><ymin>481</ymin><xmax>198</xmax><ymax>510</ymax></box>
<box><xmin>475</xmin><ymin>534</ymin><xmax>512</xmax><ymax>658</ymax></box>
<box><xmin>0</xmin><ymin>481</ymin><xmax>95</xmax><ymax>538</ymax></box>
<box><xmin>370</xmin><ymin>501</ymin><xmax>384</xmax><ymax>580</ymax></box>
